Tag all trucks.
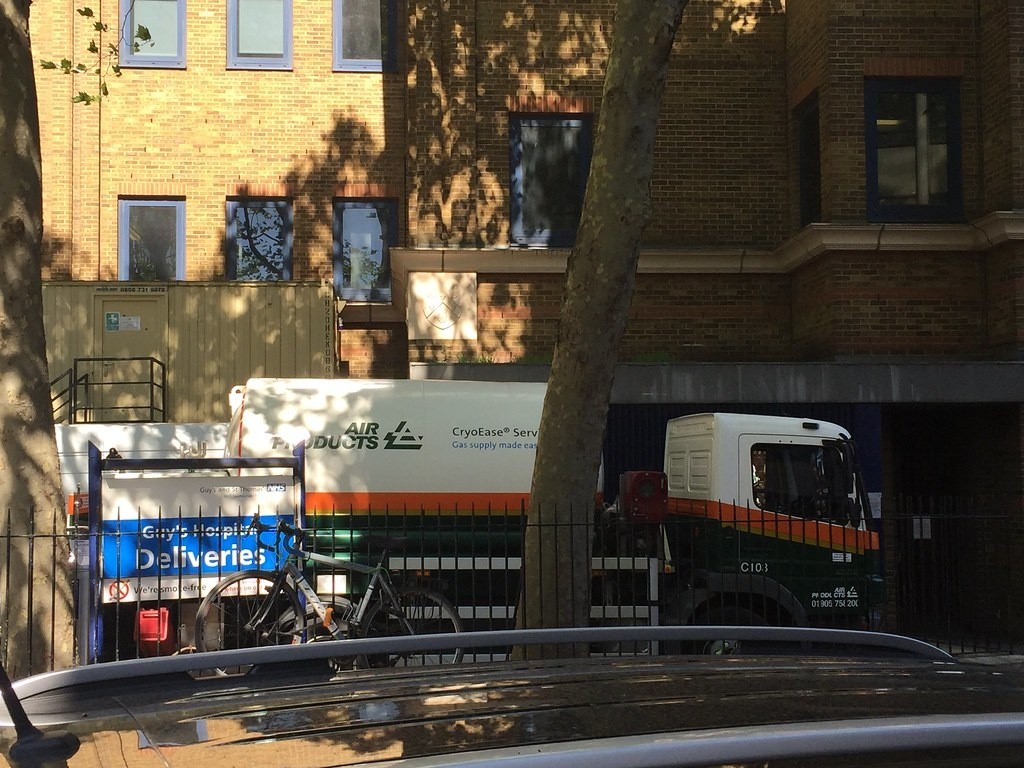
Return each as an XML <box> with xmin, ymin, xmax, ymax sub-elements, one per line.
<box><xmin>225</xmin><ymin>379</ymin><xmax>886</xmax><ymax>656</ymax></box>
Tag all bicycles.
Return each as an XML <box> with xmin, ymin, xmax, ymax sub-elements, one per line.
<box><xmin>194</xmin><ymin>519</ymin><xmax>465</xmax><ymax>664</ymax></box>
